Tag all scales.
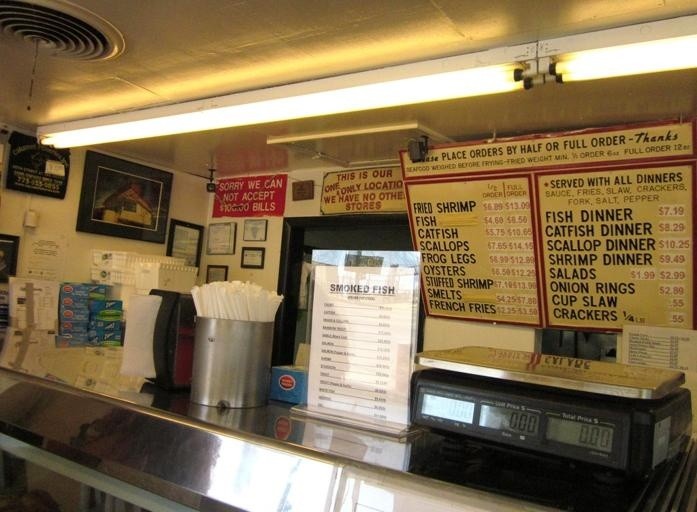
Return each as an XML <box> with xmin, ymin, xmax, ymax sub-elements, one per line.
<box><xmin>409</xmin><ymin>346</ymin><xmax>691</xmax><ymax>482</ymax></box>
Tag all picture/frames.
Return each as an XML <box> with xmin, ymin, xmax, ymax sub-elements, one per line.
<box><xmin>206</xmin><ymin>222</ymin><xmax>236</xmax><ymax>255</ymax></box>
<box><xmin>0</xmin><ymin>234</ymin><xmax>20</xmax><ymax>283</ymax></box>
<box><xmin>167</xmin><ymin>218</ymin><xmax>205</xmax><ymax>276</ymax></box>
<box><xmin>243</xmin><ymin>219</ymin><xmax>267</xmax><ymax>241</ymax></box>
<box><xmin>76</xmin><ymin>150</ymin><xmax>173</xmax><ymax>244</ymax></box>
<box><xmin>206</xmin><ymin>265</ymin><xmax>229</xmax><ymax>283</ymax></box>
<box><xmin>241</xmin><ymin>247</ymin><xmax>265</xmax><ymax>268</ymax></box>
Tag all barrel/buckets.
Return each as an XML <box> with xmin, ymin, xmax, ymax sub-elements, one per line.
<box><xmin>186</xmin><ymin>402</ymin><xmax>269</xmax><ymax>435</ymax></box>
<box><xmin>190</xmin><ymin>314</ymin><xmax>275</xmax><ymax>409</ymax></box>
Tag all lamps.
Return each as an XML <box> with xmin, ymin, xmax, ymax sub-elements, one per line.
<box><xmin>36</xmin><ymin>41</ymin><xmax>543</xmax><ymax>149</ymax></box>
<box><xmin>538</xmin><ymin>14</ymin><xmax>697</xmax><ymax>84</ymax></box>
